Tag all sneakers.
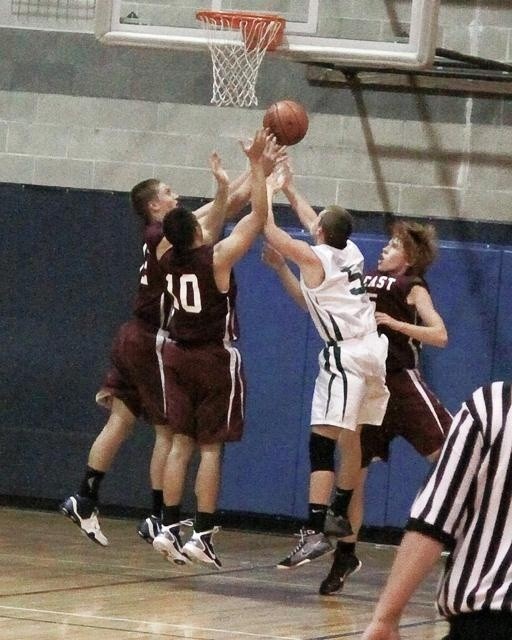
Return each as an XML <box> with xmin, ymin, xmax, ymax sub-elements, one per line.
<box><xmin>135</xmin><ymin>510</ymin><xmax>226</xmax><ymax>572</ymax></box>
<box><xmin>276</xmin><ymin>527</ymin><xmax>336</xmax><ymax>571</ymax></box>
<box><xmin>294</xmin><ymin>510</ymin><xmax>355</xmax><ymax>542</ymax></box>
<box><xmin>58</xmin><ymin>493</ymin><xmax>110</xmax><ymax>547</ymax></box>
<box><xmin>319</xmin><ymin>547</ymin><xmax>362</xmax><ymax>595</ymax></box>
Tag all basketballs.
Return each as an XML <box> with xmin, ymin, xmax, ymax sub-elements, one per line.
<box><xmin>263</xmin><ymin>99</ymin><xmax>309</xmax><ymax>145</ymax></box>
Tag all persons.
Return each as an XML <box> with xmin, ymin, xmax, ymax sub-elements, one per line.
<box><xmin>261</xmin><ymin>157</ymin><xmax>458</xmax><ymax>597</ymax></box>
<box><xmin>59</xmin><ymin>126</ymin><xmax>289</xmax><ymax>570</ymax></box>
<box><xmin>361</xmin><ymin>379</ymin><xmax>512</xmax><ymax>640</ymax></box>
